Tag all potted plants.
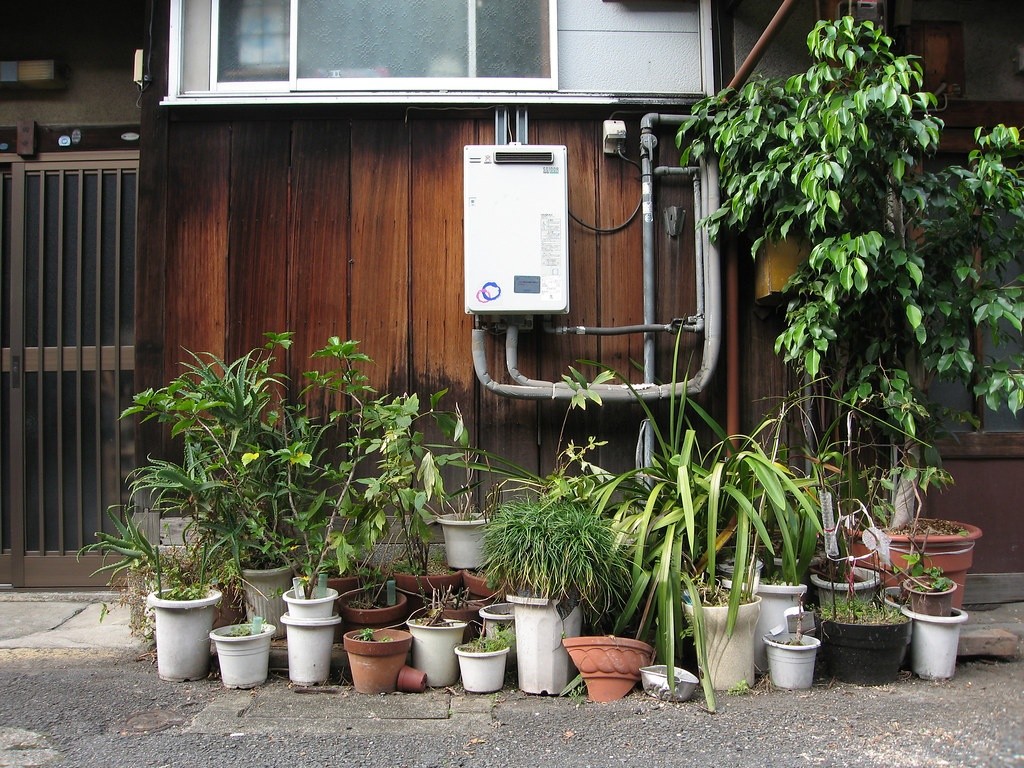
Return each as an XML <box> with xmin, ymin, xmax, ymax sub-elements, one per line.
<box><xmin>763</xmin><ymin>591</ymin><xmax>821</xmax><ymax>689</ymax></box>
<box><xmin>686</xmin><ymin>396</ymin><xmax>847</xmax><ymax>675</ymax></box>
<box><xmin>292</xmin><ymin>560</ymin><xmax>375</xmax><ymax>644</ymax></box>
<box><xmin>471</xmin><ymin>498</ymin><xmax>637</xmax><ymax>697</ymax></box>
<box><xmin>814</xmin><ymin>549</ymin><xmax>912</xmax><ymax>686</ymax></box>
<box><xmin>209</xmin><ymin>620</ymin><xmax>277</xmax><ymax>690</ymax></box>
<box><xmin>675</xmin><ymin>14</ymin><xmax>1024</xmax><ymax>610</ymax></box>
<box><xmin>479</xmin><ymin>603</ymin><xmax>516</xmax><ymax>669</ymax></box>
<box><xmin>783</xmin><ymin>411</ymin><xmax>881</xmax><ymax>608</ymax></box>
<box><xmin>175</xmin><ymin>342</ymin><xmax>298</xmax><ymax>640</ymax></box>
<box><xmin>893</xmin><ymin>554</ymin><xmax>957</xmax><ymax>616</ymax></box>
<box><xmin>329</xmin><ymin>393</ymin><xmax>462</xmax><ymax>619</ymax></box>
<box><xmin>145</xmin><ymin>567</ymin><xmax>222</xmax><ymax>683</ymax></box>
<box><xmin>454</xmin><ymin>629</ymin><xmax>517</xmax><ymax>694</ymax></box>
<box><xmin>117</xmin><ymin>331</ymin><xmax>380</xmax><ymax>622</ymax></box>
<box><xmin>343</xmin><ymin>627</ymin><xmax>412</xmax><ymax>694</ymax></box>
<box><xmin>586</xmin><ymin>418</ymin><xmax>823</xmax><ymax>715</ymax></box>
<box><xmin>405</xmin><ymin>583</ymin><xmax>467</xmax><ymax>688</ymax></box>
<box><xmin>437</xmin><ymin>402</ymin><xmax>497</xmax><ymax>570</ymax></box>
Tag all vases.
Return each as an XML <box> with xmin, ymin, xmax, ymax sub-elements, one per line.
<box><xmin>429</xmin><ymin>602</ymin><xmax>480</xmax><ymax>640</ymax></box>
<box><xmin>716</xmin><ymin>556</ymin><xmax>765</xmax><ymax>583</ymax></box>
<box><xmin>335</xmin><ymin>582</ymin><xmax>407</xmax><ymax>631</ymax></box>
<box><xmin>280</xmin><ymin>609</ymin><xmax>342</xmax><ymax>687</ymax></box>
<box><xmin>901</xmin><ymin>603</ymin><xmax>969</xmax><ymax>681</ymax></box>
<box><xmin>396</xmin><ymin>666</ymin><xmax>427</xmax><ymax>693</ymax></box>
<box><xmin>462</xmin><ymin>569</ymin><xmax>508</xmax><ymax>606</ymax></box>
